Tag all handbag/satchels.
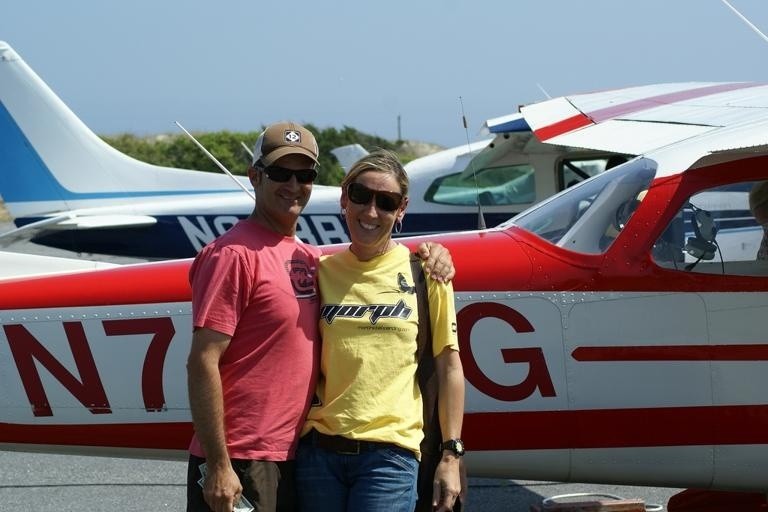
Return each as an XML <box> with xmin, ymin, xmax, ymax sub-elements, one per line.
<box><xmin>417</xmin><ymin>437</ymin><xmax>468</xmax><ymax>511</ymax></box>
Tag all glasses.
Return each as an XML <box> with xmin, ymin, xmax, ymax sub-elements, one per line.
<box><xmin>346</xmin><ymin>182</ymin><xmax>403</xmax><ymax>212</ymax></box>
<box><xmin>259</xmin><ymin>166</ymin><xmax>318</xmax><ymax>183</ymax></box>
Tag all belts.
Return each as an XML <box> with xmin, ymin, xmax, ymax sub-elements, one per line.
<box><xmin>308</xmin><ymin>429</ymin><xmax>395</xmax><ymax>455</ymax></box>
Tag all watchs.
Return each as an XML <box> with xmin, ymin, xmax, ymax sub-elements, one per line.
<box><xmin>437</xmin><ymin>437</ymin><xmax>465</xmax><ymax>459</ymax></box>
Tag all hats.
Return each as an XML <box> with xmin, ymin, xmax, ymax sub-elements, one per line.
<box><xmin>251</xmin><ymin>123</ymin><xmax>320</xmax><ymax>167</ymax></box>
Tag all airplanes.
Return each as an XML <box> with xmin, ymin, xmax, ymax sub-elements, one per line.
<box><xmin>0</xmin><ymin>35</ymin><xmax>766</xmax><ymax>510</ymax></box>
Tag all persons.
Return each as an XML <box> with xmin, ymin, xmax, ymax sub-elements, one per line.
<box><xmin>184</xmin><ymin>121</ymin><xmax>457</xmax><ymax>511</ymax></box>
<box><xmin>747</xmin><ymin>179</ymin><xmax>766</xmax><ymax>260</ymax></box>
<box><xmin>296</xmin><ymin>145</ymin><xmax>465</xmax><ymax>512</ymax></box>
